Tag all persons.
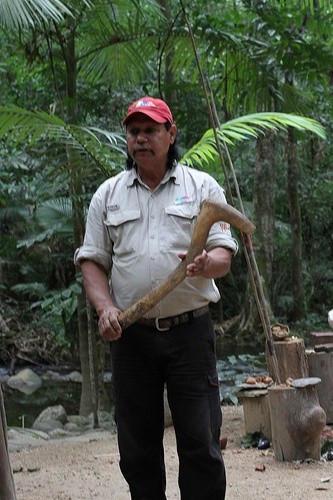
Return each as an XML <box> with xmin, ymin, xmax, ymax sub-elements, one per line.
<box><xmin>74</xmin><ymin>97</ymin><xmax>239</xmax><ymax>500</ymax></box>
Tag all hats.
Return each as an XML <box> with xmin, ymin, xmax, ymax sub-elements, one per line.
<box><xmin>123</xmin><ymin>96</ymin><xmax>173</xmax><ymax>128</ymax></box>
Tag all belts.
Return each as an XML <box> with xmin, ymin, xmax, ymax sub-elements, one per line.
<box><xmin>136</xmin><ymin>304</ymin><xmax>210</xmax><ymax>332</ymax></box>
